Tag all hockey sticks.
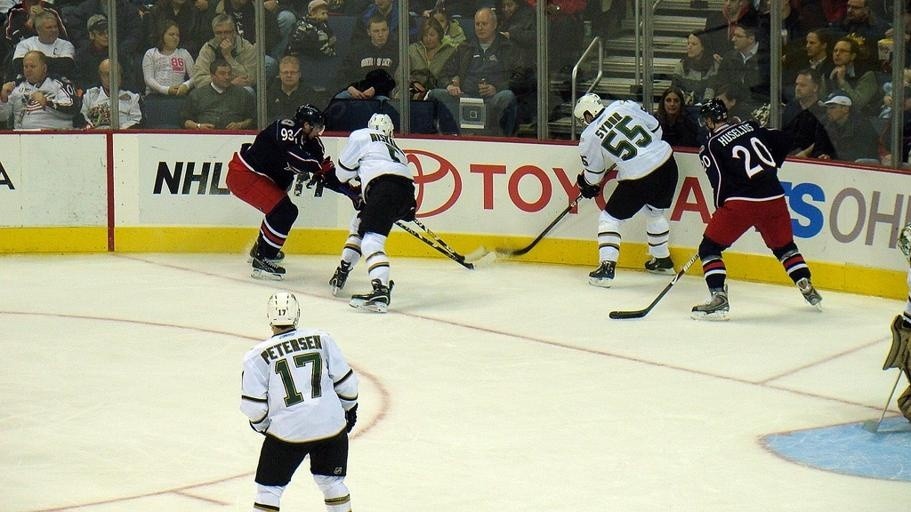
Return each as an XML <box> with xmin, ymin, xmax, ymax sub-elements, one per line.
<box><xmin>395</xmin><ymin>217</ymin><xmax>498</xmax><ymax>272</ymax></box>
<box><xmin>494</xmin><ymin>162</ymin><xmax>617</xmax><ymax>256</ymax></box>
<box><xmin>610</xmin><ymin>249</ymin><xmax>700</xmax><ymax>319</ymax></box>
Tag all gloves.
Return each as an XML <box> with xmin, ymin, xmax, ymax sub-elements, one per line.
<box><xmin>403</xmin><ymin>198</ymin><xmax>417</xmax><ymax>222</ymax></box>
<box><xmin>576</xmin><ymin>172</ymin><xmax>602</xmax><ymax>200</ymax></box>
<box><xmin>344</xmin><ymin>402</ymin><xmax>360</xmax><ymax>435</ymax></box>
<box><xmin>314</xmin><ymin>156</ymin><xmax>336</xmax><ymax>191</ymax></box>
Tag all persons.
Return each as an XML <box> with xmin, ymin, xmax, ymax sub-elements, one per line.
<box><xmin>572</xmin><ymin>91</ymin><xmax>678</xmax><ymax>280</ymax></box>
<box><xmin>692</xmin><ymin>98</ymin><xmax>822</xmax><ymax>313</ymax></box>
<box><xmin>241</xmin><ymin>289</ymin><xmax>359</xmax><ymax>512</ymax></box>
<box><xmin>882</xmin><ymin>221</ymin><xmax>911</xmax><ymax>424</ymax></box>
<box><xmin>330</xmin><ymin>112</ymin><xmax>417</xmax><ymax>306</ymax></box>
<box><xmin>225</xmin><ymin>102</ymin><xmax>335</xmax><ymax>274</ymax></box>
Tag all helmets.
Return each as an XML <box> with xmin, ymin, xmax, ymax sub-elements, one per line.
<box><xmin>266</xmin><ymin>291</ymin><xmax>301</xmax><ymax>328</ymax></box>
<box><xmin>700</xmin><ymin>97</ymin><xmax>729</xmax><ymax>124</ymax></box>
<box><xmin>291</xmin><ymin>102</ymin><xmax>326</xmax><ymax>128</ymax></box>
<box><xmin>367</xmin><ymin>111</ymin><xmax>395</xmax><ymax>136</ymax></box>
<box><xmin>572</xmin><ymin>92</ymin><xmax>606</xmax><ymax>126</ymax></box>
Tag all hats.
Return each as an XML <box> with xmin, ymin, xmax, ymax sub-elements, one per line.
<box><xmin>86</xmin><ymin>14</ymin><xmax>110</xmax><ymax>32</ymax></box>
<box><xmin>307</xmin><ymin>0</ymin><xmax>328</xmax><ymax>16</ymax></box>
<box><xmin>816</xmin><ymin>95</ymin><xmax>853</xmax><ymax>108</ymax></box>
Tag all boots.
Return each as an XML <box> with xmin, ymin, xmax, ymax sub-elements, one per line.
<box><xmin>588</xmin><ymin>260</ymin><xmax>616</xmax><ymax>279</ymax></box>
<box><xmin>796</xmin><ymin>277</ymin><xmax>823</xmax><ymax>306</ymax></box>
<box><xmin>691</xmin><ymin>283</ymin><xmax>731</xmax><ymax>313</ymax></box>
<box><xmin>328</xmin><ymin>260</ymin><xmax>353</xmax><ymax>289</ymax></box>
<box><xmin>644</xmin><ymin>255</ymin><xmax>674</xmax><ymax>271</ymax></box>
<box><xmin>351</xmin><ymin>278</ymin><xmax>394</xmax><ymax>307</ymax></box>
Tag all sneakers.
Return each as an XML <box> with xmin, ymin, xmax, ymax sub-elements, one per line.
<box><xmin>249</xmin><ymin>241</ymin><xmax>285</xmax><ymax>260</ymax></box>
<box><xmin>251</xmin><ymin>253</ymin><xmax>287</xmax><ymax>273</ymax></box>
